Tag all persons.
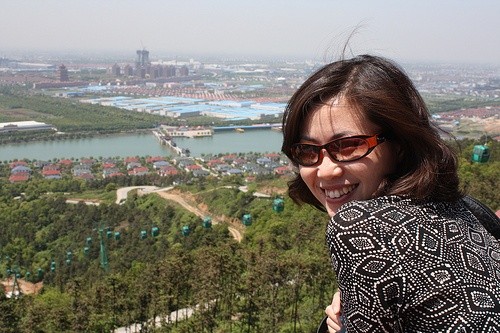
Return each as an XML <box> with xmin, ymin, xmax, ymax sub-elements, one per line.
<box><xmin>280</xmin><ymin>53</ymin><xmax>500</xmax><ymax>333</ymax></box>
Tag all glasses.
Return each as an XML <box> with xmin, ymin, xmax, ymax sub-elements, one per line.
<box><xmin>289</xmin><ymin>130</ymin><xmax>388</xmax><ymax>168</ymax></box>
